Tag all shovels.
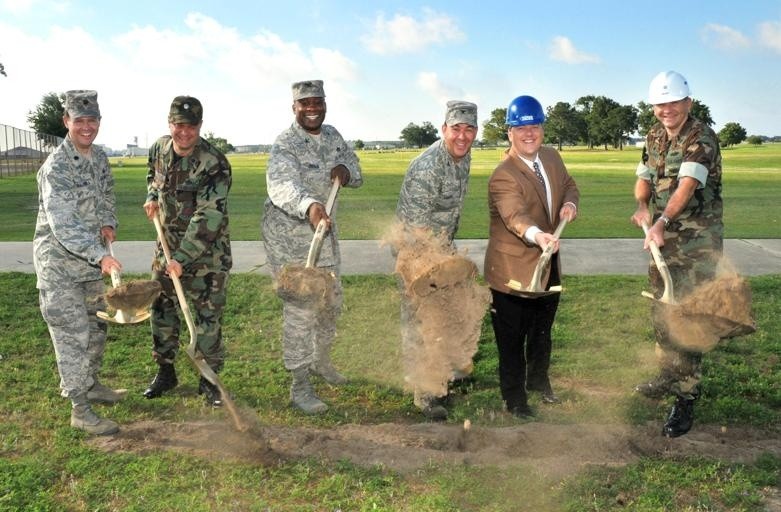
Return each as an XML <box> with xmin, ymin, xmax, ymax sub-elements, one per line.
<box><xmin>152</xmin><ymin>209</ymin><xmax>219</xmax><ymax>385</ymax></box>
<box><xmin>276</xmin><ymin>175</ymin><xmax>342</xmax><ymax>310</ymax></box>
<box><xmin>641</xmin><ymin>218</ymin><xmax>681</xmax><ymax>307</ymax></box>
<box><xmin>504</xmin><ymin>212</ymin><xmax>569</xmax><ymax>298</ymax></box>
<box><xmin>96</xmin><ymin>235</ymin><xmax>152</xmax><ymax>324</ymax></box>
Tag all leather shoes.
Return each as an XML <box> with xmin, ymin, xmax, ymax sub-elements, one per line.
<box><xmin>414</xmin><ymin>377</ymin><xmax>472</xmax><ymax>419</ymax></box>
<box><xmin>507</xmin><ymin>384</ymin><xmax>560</xmax><ymax>420</ymax></box>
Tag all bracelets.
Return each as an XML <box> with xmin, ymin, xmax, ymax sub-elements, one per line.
<box><xmin>658</xmin><ymin>214</ymin><xmax>671</xmax><ymax>224</ymax></box>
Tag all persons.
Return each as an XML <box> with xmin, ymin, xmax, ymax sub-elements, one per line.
<box><xmin>389</xmin><ymin>99</ymin><xmax>479</xmax><ymax>419</ymax></box>
<box><xmin>631</xmin><ymin>70</ymin><xmax>725</xmax><ymax>437</ymax></box>
<box><xmin>261</xmin><ymin>78</ymin><xmax>363</xmax><ymax>415</ymax></box>
<box><xmin>33</xmin><ymin>89</ymin><xmax>129</xmax><ymax>435</ymax></box>
<box><xmin>142</xmin><ymin>96</ymin><xmax>233</xmax><ymax>407</ymax></box>
<box><xmin>483</xmin><ymin>93</ymin><xmax>582</xmax><ymax>410</ymax></box>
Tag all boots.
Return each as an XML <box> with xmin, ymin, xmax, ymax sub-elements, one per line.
<box><xmin>636</xmin><ymin>366</ymin><xmax>697</xmax><ymax>439</ymax></box>
<box><xmin>145</xmin><ymin>365</ymin><xmax>177</xmax><ymax>397</ymax></box>
<box><xmin>198</xmin><ymin>372</ymin><xmax>222</xmax><ymax>405</ymax></box>
<box><xmin>290</xmin><ymin>377</ymin><xmax>329</xmax><ymax>413</ymax></box>
<box><xmin>310</xmin><ymin>351</ymin><xmax>349</xmax><ymax>386</ymax></box>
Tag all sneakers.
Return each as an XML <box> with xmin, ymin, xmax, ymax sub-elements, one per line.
<box><xmin>87</xmin><ymin>383</ymin><xmax>127</xmax><ymax>402</ymax></box>
<box><xmin>70</xmin><ymin>410</ymin><xmax>117</xmax><ymax>435</ymax></box>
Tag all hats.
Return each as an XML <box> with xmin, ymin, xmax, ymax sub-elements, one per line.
<box><xmin>291</xmin><ymin>81</ymin><xmax>324</xmax><ymax>102</ymax></box>
<box><xmin>169</xmin><ymin>96</ymin><xmax>203</xmax><ymax>125</ymax></box>
<box><xmin>445</xmin><ymin>101</ymin><xmax>478</xmax><ymax>127</ymax></box>
<box><xmin>65</xmin><ymin>90</ymin><xmax>101</xmax><ymax>122</ymax></box>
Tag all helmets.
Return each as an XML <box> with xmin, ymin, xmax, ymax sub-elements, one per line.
<box><xmin>506</xmin><ymin>96</ymin><xmax>545</xmax><ymax>126</ymax></box>
<box><xmin>648</xmin><ymin>70</ymin><xmax>690</xmax><ymax>105</ymax></box>
